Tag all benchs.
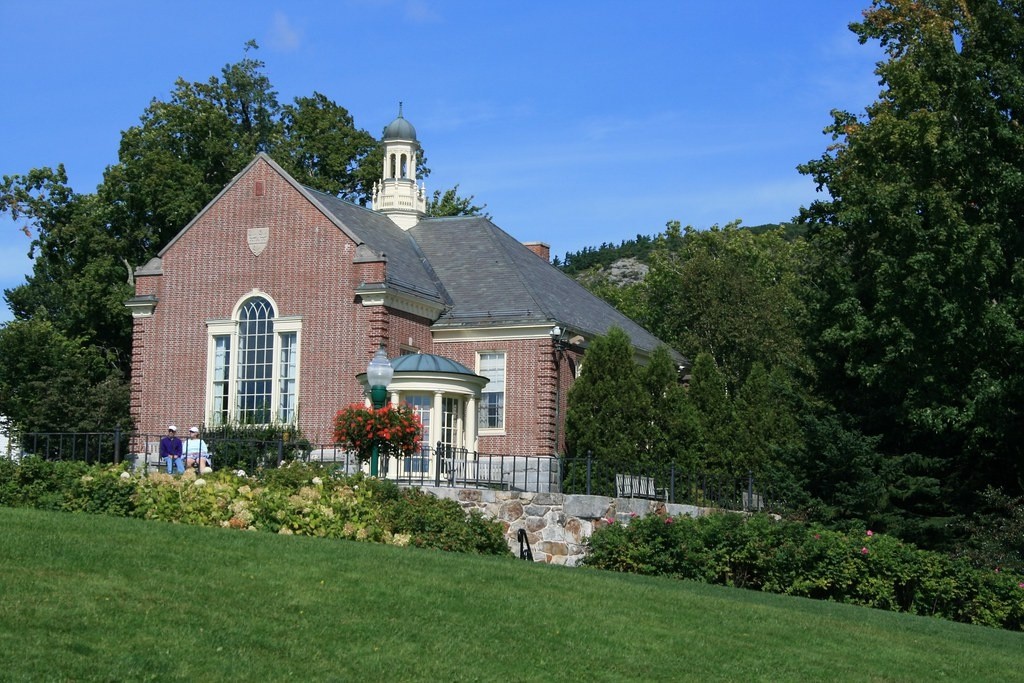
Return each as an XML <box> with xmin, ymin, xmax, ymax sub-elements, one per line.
<box><xmin>144</xmin><ymin>441</ymin><xmax>212</xmax><ymax>472</ymax></box>
<box><xmin>743</xmin><ymin>492</ymin><xmax>764</xmax><ymax>512</ymax></box>
<box><xmin>616</xmin><ymin>474</ymin><xmax>669</xmax><ymax>503</ymax></box>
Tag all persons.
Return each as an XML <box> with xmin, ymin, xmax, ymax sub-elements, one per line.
<box><xmin>160</xmin><ymin>425</ymin><xmax>185</xmax><ymax>474</ymax></box>
<box><xmin>182</xmin><ymin>426</ymin><xmax>209</xmax><ymax>471</ymax></box>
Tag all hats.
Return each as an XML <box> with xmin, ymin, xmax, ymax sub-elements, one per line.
<box><xmin>189</xmin><ymin>427</ymin><xmax>199</xmax><ymax>433</ymax></box>
<box><xmin>169</xmin><ymin>426</ymin><xmax>177</xmax><ymax>432</ymax></box>
